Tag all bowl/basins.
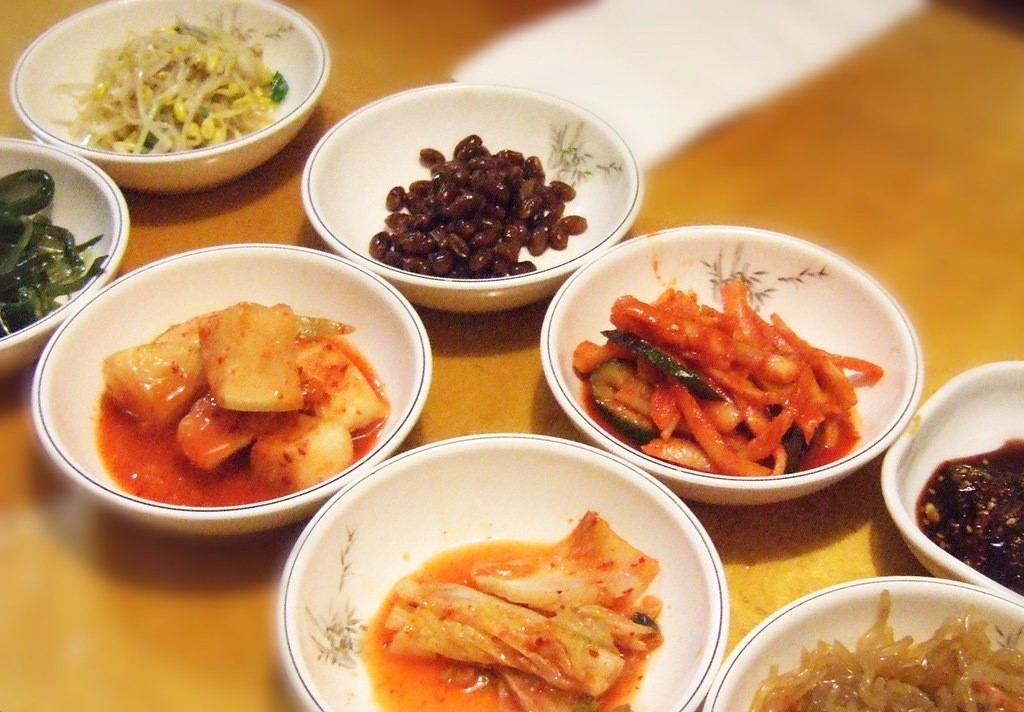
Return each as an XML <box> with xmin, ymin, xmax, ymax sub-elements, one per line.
<box><xmin>299</xmin><ymin>81</ymin><xmax>646</xmax><ymax>313</ymax></box>
<box><xmin>32</xmin><ymin>242</ymin><xmax>434</xmax><ymax>537</ymax></box>
<box><xmin>701</xmin><ymin>575</ymin><xmax>1024</xmax><ymax>712</ymax></box>
<box><xmin>0</xmin><ymin>136</ymin><xmax>130</xmax><ymax>380</ymax></box>
<box><xmin>539</xmin><ymin>224</ymin><xmax>925</xmax><ymax>506</ymax></box>
<box><xmin>9</xmin><ymin>0</ymin><xmax>331</xmax><ymax>196</ymax></box>
<box><xmin>277</xmin><ymin>431</ymin><xmax>730</xmax><ymax>712</ymax></box>
<box><xmin>880</xmin><ymin>362</ymin><xmax>1024</xmax><ymax>601</ymax></box>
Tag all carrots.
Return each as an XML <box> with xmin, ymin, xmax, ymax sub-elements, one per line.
<box><xmin>609</xmin><ymin>281</ymin><xmax>885</xmax><ymax>476</ymax></box>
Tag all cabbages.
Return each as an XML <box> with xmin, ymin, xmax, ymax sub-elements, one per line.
<box><xmin>383</xmin><ymin>510</ymin><xmax>662</xmax><ymax>712</ymax></box>
